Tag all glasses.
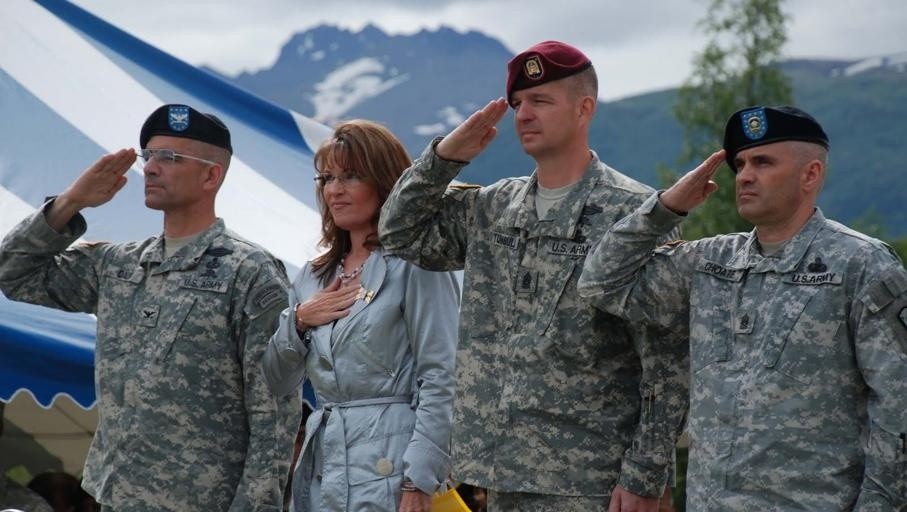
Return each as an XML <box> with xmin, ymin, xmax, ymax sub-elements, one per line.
<box><xmin>313</xmin><ymin>171</ymin><xmax>363</xmax><ymax>189</ymax></box>
<box><xmin>135</xmin><ymin>147</ymin><xmax>220</xmax><ymax>168</ymax></box>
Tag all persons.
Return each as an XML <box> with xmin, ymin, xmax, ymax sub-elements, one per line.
<box><xmin>261</xmin><ymin>119</ymin><xmax>460</xmax><ymax>512</ymax></box>
<box><xmin>377</xmin><ymin>40</ymin><xmax>690</xmax><ymax>512</ymax></box>
<box><xmin>576</xmin><ymin>105</ymin><xmax>906</xmax><ymax>512</ymax></box>
<box><xmin>0</xmin><ymin>103</ymin><xmax>304</xmax><ymax>512</ymax></box>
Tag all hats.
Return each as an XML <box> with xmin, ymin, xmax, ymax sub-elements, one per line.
<box><xmin>506</xmin><ymin>40</ymin><xmax>591</xmax><ymax>107</ymax></box>
<box><xmin>724</xmin><ymin>105</ymin><xmax>829</xmax><ymax>173</ymax></box>
<box><xmin>139</xmin><ymin>104</ymin><xmax>234</xmax><ymax>149</ymax></box>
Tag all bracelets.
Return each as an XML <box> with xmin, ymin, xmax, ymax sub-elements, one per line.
<box><xmin>293</xmin><ymin>301</ymin><xmax>311</xmax><ymax>329</ymax></box>
<box><xmin>398</xmin><ymin>480</ymin><xmax>422</xmax><ymax>493</ymax></box>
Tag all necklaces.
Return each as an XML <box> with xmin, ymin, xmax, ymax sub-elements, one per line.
<box><xmin>336</xmin><ymin>250</ymin><xmax>374</xmax><ymax>285</ymax></box>
